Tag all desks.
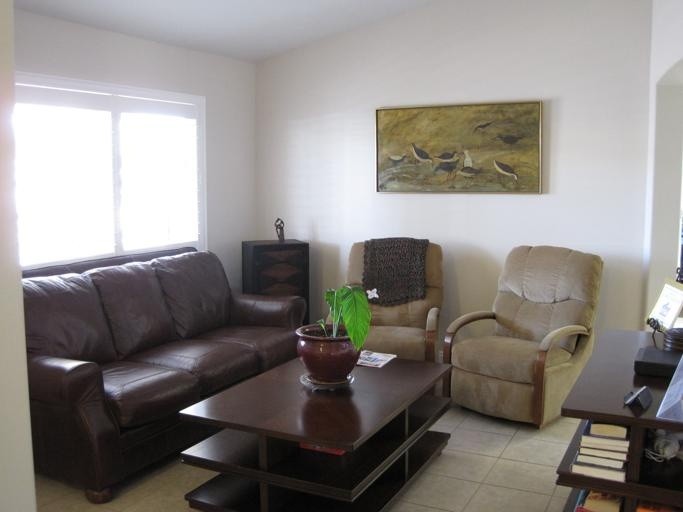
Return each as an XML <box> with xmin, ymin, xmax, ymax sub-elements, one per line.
<box><xmin>553</xmin><ymin>326</ymin><xmax>680</xmax><ymax>512</ymax></box>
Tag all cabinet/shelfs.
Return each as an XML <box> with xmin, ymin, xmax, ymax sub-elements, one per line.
<box><xmin>242</xmin><ymin>239</ymin><xmax>310</xmax><ymax>327</ymax></box>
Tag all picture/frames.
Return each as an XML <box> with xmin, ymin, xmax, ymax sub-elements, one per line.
<box><xmin>374</xmin><ymin>101</ymin><xmax>543</xmax><ymax>196</ymax></box>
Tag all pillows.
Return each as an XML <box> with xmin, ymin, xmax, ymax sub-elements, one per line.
<box><xmin>19</xmin><ymin>252</ymin><xmax>235</xmax><ymax>365</ymax></box>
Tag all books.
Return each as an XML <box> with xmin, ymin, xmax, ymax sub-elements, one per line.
<box><xmin>356</xmin><ymin>349</ymin><xmax>398</xmax><ymax>368</ymax></box>
<box><xmin>647</xmin><ymin>279</ymin><xmax>683</xmax><ymax>330</ymax></box>
<box><xmin>568</xmin><ymin>419</ymin><xmax>679</xmax><ymax>512</ymax></box>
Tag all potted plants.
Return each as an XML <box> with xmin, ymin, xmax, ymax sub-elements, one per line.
<box><xmin>295</xmin><ymin>282</ymin><xmax>373</xmax><ymax>394</ymax></box>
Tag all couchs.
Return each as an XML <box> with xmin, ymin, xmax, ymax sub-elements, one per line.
<box><xmin>17</xmin><ymin>244</ymin><xmax>307</xmax><ymax>501</ymax></box>
<box><xmin>328</xmin><ymin>237</ymin><xmax>444</xmax><ymax>361</ymax></box>
<box><xmin>444</xmin><ymin>245</ymin><xmax>603</xmax><ymax>432</ymax></box>
